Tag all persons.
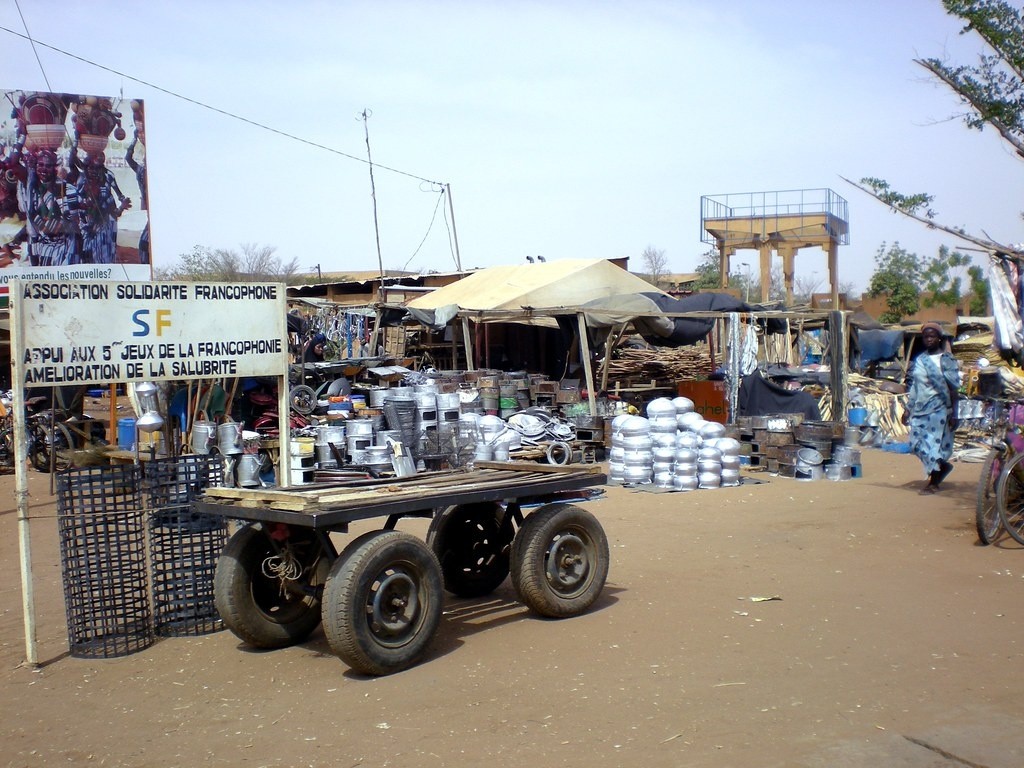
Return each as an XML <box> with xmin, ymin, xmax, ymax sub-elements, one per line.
<box><xmin>297</xmin><ymin>337</ymin><xmax>324</xmax><ymax>363</ymax></box>
<box><xmin>1</xmin><ymin>119</ymin><xmax>148</xmax><ymax>265</ymax></box>
<box><xmin>902</xmin><ymin>323</ymin><xmax>961</xmax><ymax>495</ymax></box>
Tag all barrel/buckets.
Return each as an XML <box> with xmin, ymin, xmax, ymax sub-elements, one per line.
<box><xmin>193</xmin><ymin>409</ymin><xmax>216</xmax><ymax>455</ymax></box>
<box><xmin>177</xmin><ymin>444</ymin><xmax>201</xmax><ymax>487</ymax></box>
<box><xmin>234</xmin><ymin>453</ymin><xmax>262</xmax><ymax>487</ymax></box>
<box><xmin>217</xmin><ymin>414</ymin><xmax>245</xmax><ymax>455</ymax></box>
<box><xmin>207</xmin><ymin>446</ymin><xmax>234</xmax><ymax>488</ymax></box>
<box><xmin>118</xmin><ymin>418</ymin><xmax>135</xmax><ymax>451</ymax></box>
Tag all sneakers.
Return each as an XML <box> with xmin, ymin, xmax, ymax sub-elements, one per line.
<box><xmin>935</xmin><ymin>462</ymin><xmax>954</xmax><ymax>486</ymax></box>
<box><xmin>919</xmin><ymin>482</ymin><xmax>940</xmax><ymax>495</ymax></box>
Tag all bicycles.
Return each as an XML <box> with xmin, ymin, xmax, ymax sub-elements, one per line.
<box><xmin>962</xmin><ymin>360</ymin><xmax>1024</xmax><ymax>546</ymax></box>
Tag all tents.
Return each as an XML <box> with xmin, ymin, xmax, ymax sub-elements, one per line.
<box><xmin>404</xmin><ymin>258</ymin><xmax>681</xmax><ymax>417</ymax></box>
